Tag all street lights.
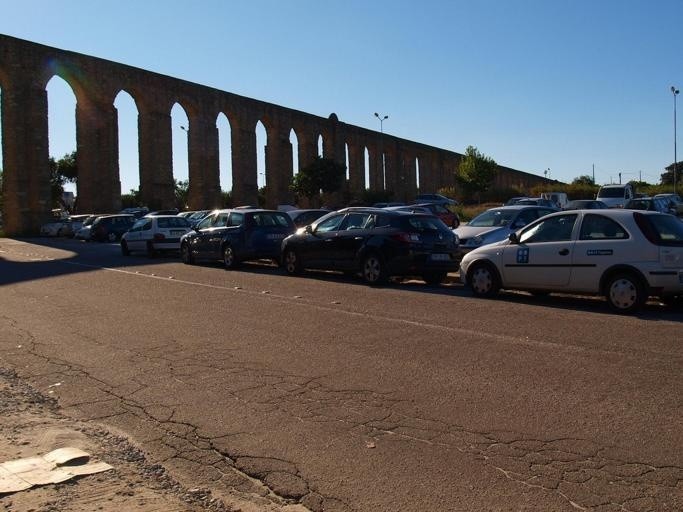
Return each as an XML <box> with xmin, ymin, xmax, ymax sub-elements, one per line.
<box><xmin>671</xmin><ymin>85</ymin><xmax>679</xmax><ymax>192</ymax></box>
<box><xmin>374</xmin><ymin>112</ymin><xmax>388</xmax><ymax>133</ymax></box>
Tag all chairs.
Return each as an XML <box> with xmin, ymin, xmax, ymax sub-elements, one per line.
<box><xmin>583</xmin><ymin>223</ymin><xmax>617</xmax><ymax>239</ymax></box>
